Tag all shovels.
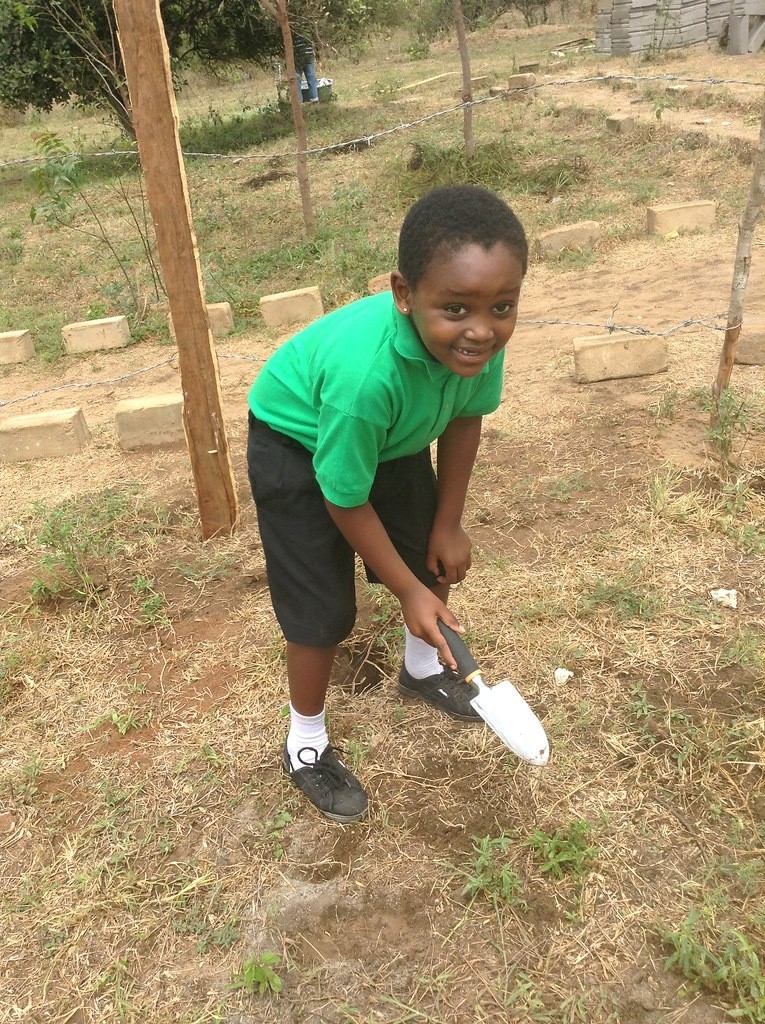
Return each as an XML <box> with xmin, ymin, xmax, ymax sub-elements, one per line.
<box><xmin>436</xmin><ymin>617</ymin><xmax>550</xmax><ymax>768</ymax></box>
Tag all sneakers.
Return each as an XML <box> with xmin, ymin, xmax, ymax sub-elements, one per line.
<box><xmin>398</xmin><ymin>657</ymin><xmax>485</xmax><ymax>722</ymax></box>
<box><xmin>282</xmin><ymin>734</ymin><xmax>369</xmax><ymax>823</ymax></box>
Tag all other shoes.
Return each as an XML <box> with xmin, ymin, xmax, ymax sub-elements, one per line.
<box><xmin>310</xmin><ymin>97</ymin><xmax>319</xmax><ymax>103</ymax></box>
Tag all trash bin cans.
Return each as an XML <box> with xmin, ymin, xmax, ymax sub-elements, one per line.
<box><xmin>300</xmin><ymin>78</ymin><xmax>332</xmax><ymax>103</ymax></box>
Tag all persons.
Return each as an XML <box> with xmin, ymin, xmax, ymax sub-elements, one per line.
<box><xmin>293</xmin><ymin>30</ymin><xmax>323</xmax><ymax>105</ymax></box>
<box><xmin>238</xmin><ymin>182</ymin><xmax>530</xmax><ymax>831</ymax></box>
